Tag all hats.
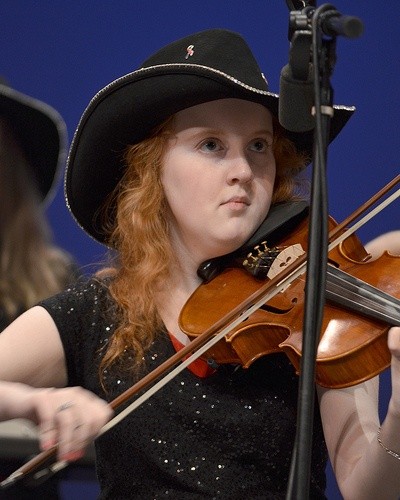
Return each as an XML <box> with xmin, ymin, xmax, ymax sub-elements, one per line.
<box><xmin>0</xmin><ymin>83</ymin><xmax>67</xmax><ymax>212</ymax></box>
<box><xmin>64</xmin><ymin>31</ymin><xmax>355</xmax><ymax>249</ymax></box>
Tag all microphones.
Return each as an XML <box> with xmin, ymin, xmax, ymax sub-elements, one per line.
<box><xmin>279</xmin><ymin>28</ymin><xmax>319</xmax><ymax>133</ymax></box>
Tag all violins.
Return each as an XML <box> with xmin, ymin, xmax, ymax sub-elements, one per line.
<box><xmin>177</xmin><ymin>196</ymin><xmax>400</xmax><ymax>388</ymax></box>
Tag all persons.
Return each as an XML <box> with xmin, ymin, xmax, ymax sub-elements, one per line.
<box><xmin>0</xmin><ymin>28</ymin><xmax>400</xmax><ymax>500</ymax></box>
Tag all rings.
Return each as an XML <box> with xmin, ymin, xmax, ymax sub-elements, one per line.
<box><xmin>56</xmin><ymin>402</ymin><xmax>74</xmax><ymax>413</ymax></box>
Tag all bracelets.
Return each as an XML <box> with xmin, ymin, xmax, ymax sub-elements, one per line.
<box><xmin>377</xmin><ymin>426</ymin><xmax>400</xmax><ymax>461</ymax></box>
